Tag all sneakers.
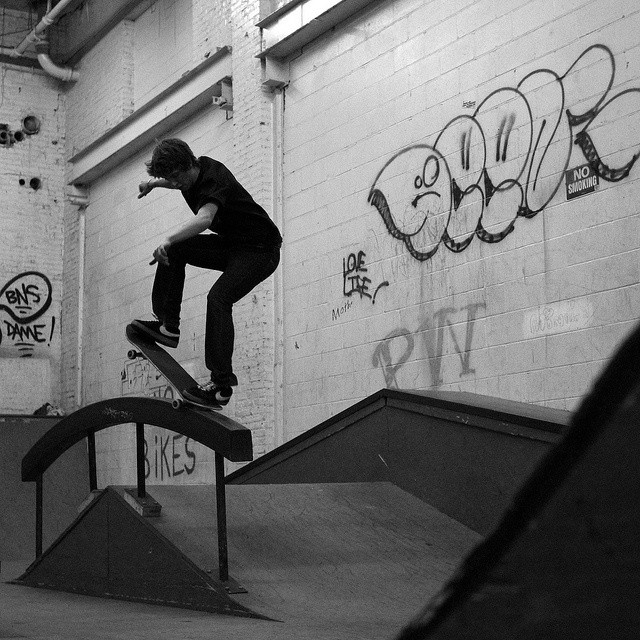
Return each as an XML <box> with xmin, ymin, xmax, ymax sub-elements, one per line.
<box><xmin>183</xmin><ymin>381</ymin><xmax>232</xmax><ymax>406</ymax></box>
<box><xmin>132</xmin><ymin>319</ymin><xmax>180</xmax><ymax>348</ymax></box>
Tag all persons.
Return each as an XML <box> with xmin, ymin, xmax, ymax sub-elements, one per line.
<box><xmin>131</xmin><ymin>138</ymin><xmax>283</xmax><ymax>405</ymax></box>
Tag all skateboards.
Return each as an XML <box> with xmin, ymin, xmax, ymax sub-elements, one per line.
<box><xmin>125</xmin><ymin>324</ymin><xmax>223</xmax><ymax>410</ymax></box>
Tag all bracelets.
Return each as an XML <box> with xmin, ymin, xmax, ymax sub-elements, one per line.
<box><xmin>147</xmin><ymin>177</ymin><xmax>153</xmax><ymax>190</ymax></box>
